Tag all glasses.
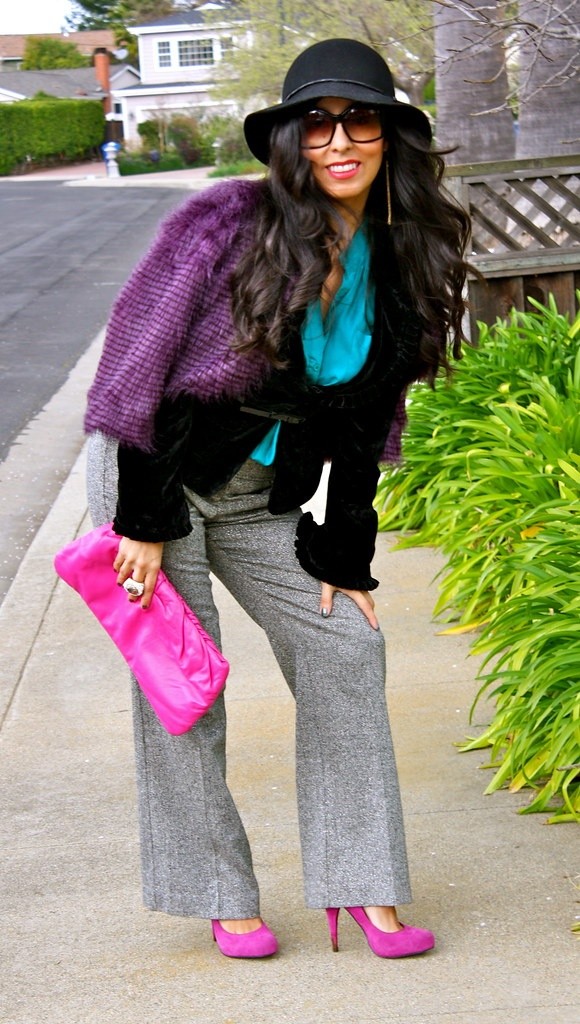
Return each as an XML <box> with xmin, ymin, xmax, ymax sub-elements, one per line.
<box><xmin>290</xmin><ymin>103</ymin><xmax>388</xmax><ymax>149</ymax></box>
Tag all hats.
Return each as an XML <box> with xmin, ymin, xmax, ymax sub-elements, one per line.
<box><xmin>243</xmin><ymin>38</ymin><xmax>430</xmax><ymax>169</ymax></box>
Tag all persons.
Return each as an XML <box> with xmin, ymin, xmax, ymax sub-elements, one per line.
<box><xmin>81</xmin><ymin>40</ymin><xmax>474</xmax><ymax>956</ymax></box>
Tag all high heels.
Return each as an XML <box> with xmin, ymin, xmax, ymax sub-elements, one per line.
<box><xmin>212</xmin><ymin>919</ymin><xmax>278</xmax><ymax>959</ymax></box>
<box><xmin>325</xmin><ymin>907</ymin><xmax>435</xmax><ymax>960</ymax></box>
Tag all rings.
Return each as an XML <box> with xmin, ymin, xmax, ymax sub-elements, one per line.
<box><xmin>123</xmin><ymin>578</ymin><xmax>145</xmax><ymax>596</ymax></box>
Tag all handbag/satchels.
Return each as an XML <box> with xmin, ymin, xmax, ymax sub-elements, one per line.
<box><xmin>54</xmin><ymin>524</ymin><xmax>230</xmax><ymax>737</ymax></box>
